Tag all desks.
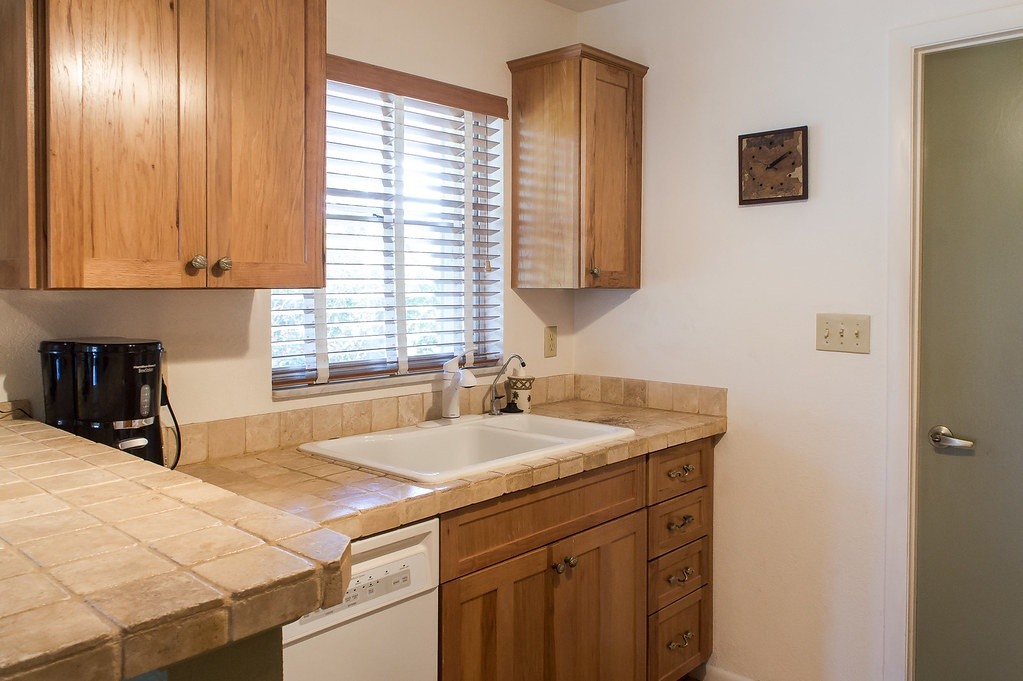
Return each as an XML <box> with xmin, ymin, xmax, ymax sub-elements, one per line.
<box><xmin>0</xmin><ymin>399</ymin><xmax>351</xmax><ymax>681</ymax></box>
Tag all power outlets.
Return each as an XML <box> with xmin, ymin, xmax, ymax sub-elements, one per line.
<box><xmin>544</xmin><ymin>325</ymin><xmax>558</xmax><ymax>357</ymax></box>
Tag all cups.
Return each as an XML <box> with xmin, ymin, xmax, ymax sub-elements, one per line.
<box><xmin>507</xmin><ymin>375</ymin><xmax>535</xmax><ymax>412</ymax></box>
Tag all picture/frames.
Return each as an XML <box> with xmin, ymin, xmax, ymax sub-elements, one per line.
<box><xmin>738</xmin><ymin>125</ymin><xmax>808</xmax><ymax>206</ymax></box>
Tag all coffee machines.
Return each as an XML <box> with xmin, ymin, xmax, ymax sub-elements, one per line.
<box><xmin>37</xmin><ymin>337</ymin><xmax>165</xmax><ymax>470</ymax></box>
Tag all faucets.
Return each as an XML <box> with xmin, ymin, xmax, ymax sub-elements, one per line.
<box><xmin>488</xmin><ymin>354</ymin><xmax>527</xmax><ymax>416</ymax></box>
<box><xmin>443</xmin><ymin>347</ymin><xmax>478</xmax><ymax>419</ymax></box>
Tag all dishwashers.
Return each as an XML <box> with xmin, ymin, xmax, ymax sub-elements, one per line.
<box><xmin>281</xmin><ymin>518</ymin><xmax>440</xmax><ymax>681</ymax></box>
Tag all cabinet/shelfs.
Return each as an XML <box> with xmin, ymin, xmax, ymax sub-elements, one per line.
<box><xmin>506</xmin><ymin>43</ymin><xmax>650</xmax><ymax>289</ymax></box>
<box><xmin>439</xmin><ymin>437</ymin><xmax>713</xmax><ymax>681</ymax></box>
<box><xmin>0</xmin><ymin>0</ymin><xmax>326</xmax><ymax>290</ymax></box>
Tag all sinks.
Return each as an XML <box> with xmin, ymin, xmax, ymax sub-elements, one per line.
<box><xmin>477</xmin><ymin>414</ymin><xmax>636</xmax><ymax>441</ymax></box>
<box><xmin>296</xmin><ymin>424</ymin><xmax>569</xmax><ymax>486</ymax></box>
